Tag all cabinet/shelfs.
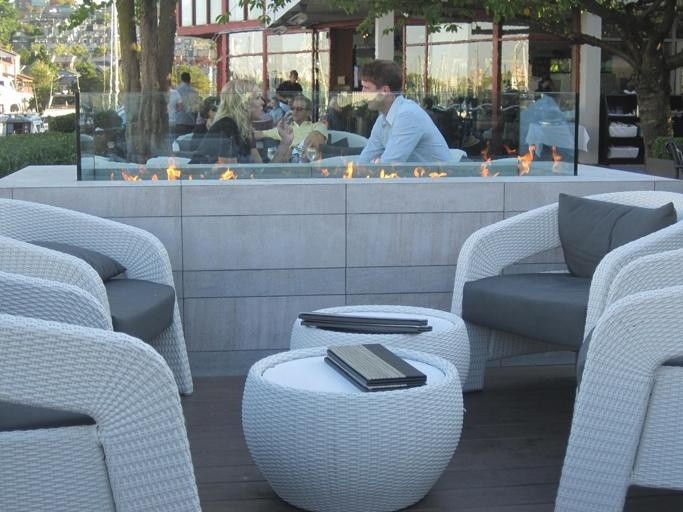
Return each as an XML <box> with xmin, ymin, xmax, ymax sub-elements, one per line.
<box><xmin>601</xmin><ymin>88</ymin><xmax>647</xmax><ymax>165</ymax></box>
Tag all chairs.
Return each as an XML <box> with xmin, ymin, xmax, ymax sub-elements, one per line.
<box><xmin>664</xmin><ymin>141</ymin><xmax>682</xmax><ymax>179</ymax></box>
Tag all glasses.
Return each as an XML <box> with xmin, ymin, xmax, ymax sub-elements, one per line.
<box><xmin>290</xmin><ymin>105</ymin><xmax>308</xmax><ymax>113</ymax></box>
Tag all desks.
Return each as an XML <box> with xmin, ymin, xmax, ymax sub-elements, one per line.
<box><xmin>525</xmin><ymin>121</ymin><xmax>589</xmax><ymax>165</ymax></box>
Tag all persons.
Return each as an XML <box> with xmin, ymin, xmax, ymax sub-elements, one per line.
<box><xmin>276</xmin><ymin>70</ymin><xmax>303</xmax><ymax>112</ymax></box>
<box><xmin>356</xmin><ymin>59</ymin><xmax>458</xmax><ymax>165</ymax></box>
<box><xmin>266</xmin><ymin>97</ymin><xmax>285</xmax><ymax>126</ymax></box>
<box><xmin>326</xmin><ymin>94</ymin><xmax>378</xmax><ymax>136</ymax></box>
<box><xmin>255</xmin><ymin>95</ymin><xmax>328</xmax><ymax>162</ymax></box>
<box><xmin>188</xmin><ymin>96</ymin><xmax>221</xmax><ymax>151</ymax></box>
<box><xmin>176</xmin><ymin>72</ymin><xmax>200</xmax><ymax>138</ymax></box>
<box><xmin>167</xmin><ymin>89</ymin><xmax>182</xmax><ymax>133</ymax></box>
<box><xmin>503</xmin><ymin>76</ymin><xmax>583</xmax><ymax>160</ymax></box>
<box><xmin>420</xmin><ymin>94</ymin><xmax>471</xmax><ymax>138</ymax></box>
<box><xmin>186</xmin><ymin>80</ymin><xmax>293</xmax><ymax>164</ymax></box>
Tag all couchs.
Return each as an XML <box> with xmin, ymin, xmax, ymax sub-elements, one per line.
<box><xmin>450</xmin><ymin>189</ymin><xmax>683</xmax><ymax>393</ymax></box>
<box><xmin>0</xmin><ymin>198</ymin><xmax>198</xmax><ymax>396</ymax></box>
<box><xmin>2</xmin><ymin>270</ymin><xmax>202</xmax><ymax>512</ymax></box>
<box><xmin>173</xmin><ymin>133</ymin><xmax>195</xmax><ymax>153</ymax></box>
<box><xmin>551</xmin><ymin>247</ymin><xmax>682</xmax><ymax>512</ymax></box>
<box><xmin>324</xmin><ymin>129</ymin><xmax>370</xmax><ymax>156</ymax></box>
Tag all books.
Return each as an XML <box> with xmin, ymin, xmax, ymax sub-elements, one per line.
<box><xmin>325</xmin><ymin>342</ymin><xmax>426</xmax><ymax>393</ymax></box>
<box><xmin>299</xmin><ymin>310</ymin><xmax>433</xmax><ymax>332</ymax></box>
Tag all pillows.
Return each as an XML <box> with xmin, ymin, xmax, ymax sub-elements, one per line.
<box><xmin>321</xmin><ymin>144</ymin><xmax>364</xmax><ymax>159</ymax></box>
<box><xmin>558</xmin><ymin>191</ymin><xmax>677</xmax><ymax>277</ymax></box>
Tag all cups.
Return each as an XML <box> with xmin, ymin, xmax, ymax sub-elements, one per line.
<box><xmin>267</xmin><ymin>146</ymin><xmax>277</xmax><ymax>161</ymax></box>
<box><xmin>306</xmin><ymin>147</ymin><xmax>316</xmax><ymax>163</ymax></box>
